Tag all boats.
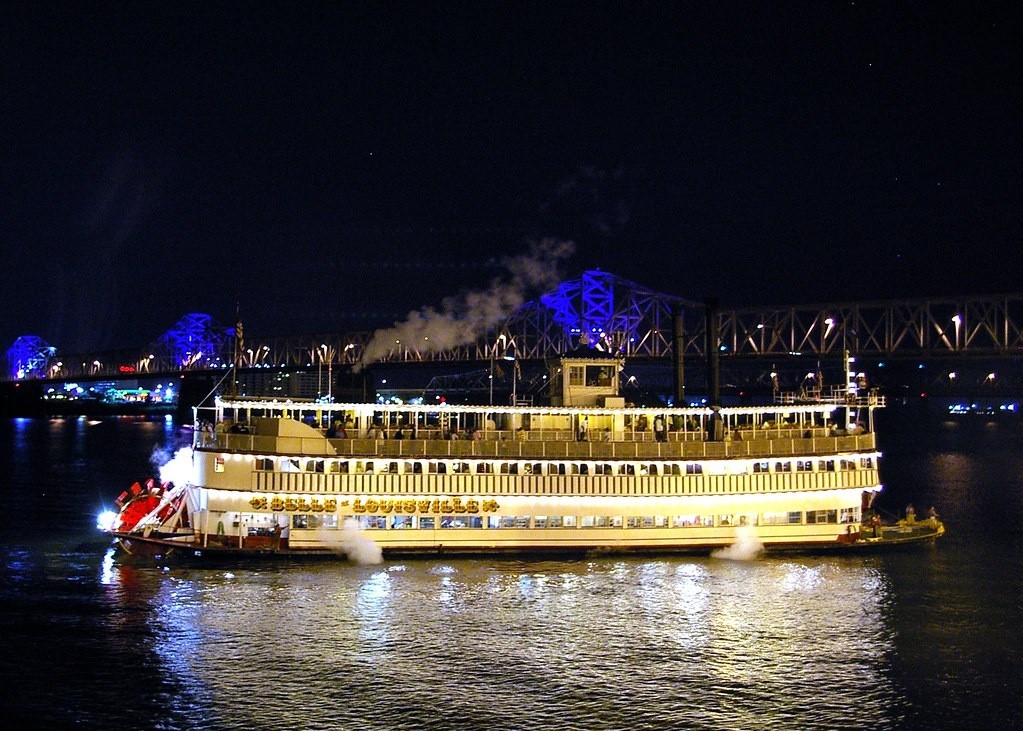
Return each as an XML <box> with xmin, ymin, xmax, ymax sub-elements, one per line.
<box><xmin>97</xmin><ymin>353</ymin><xmax>885</xmax><ymax>561</ymax></box>
<box><xmin>832</xmin><ymin>518</ymin><xmax>943</xmax><ymax>550</ymax></box>
